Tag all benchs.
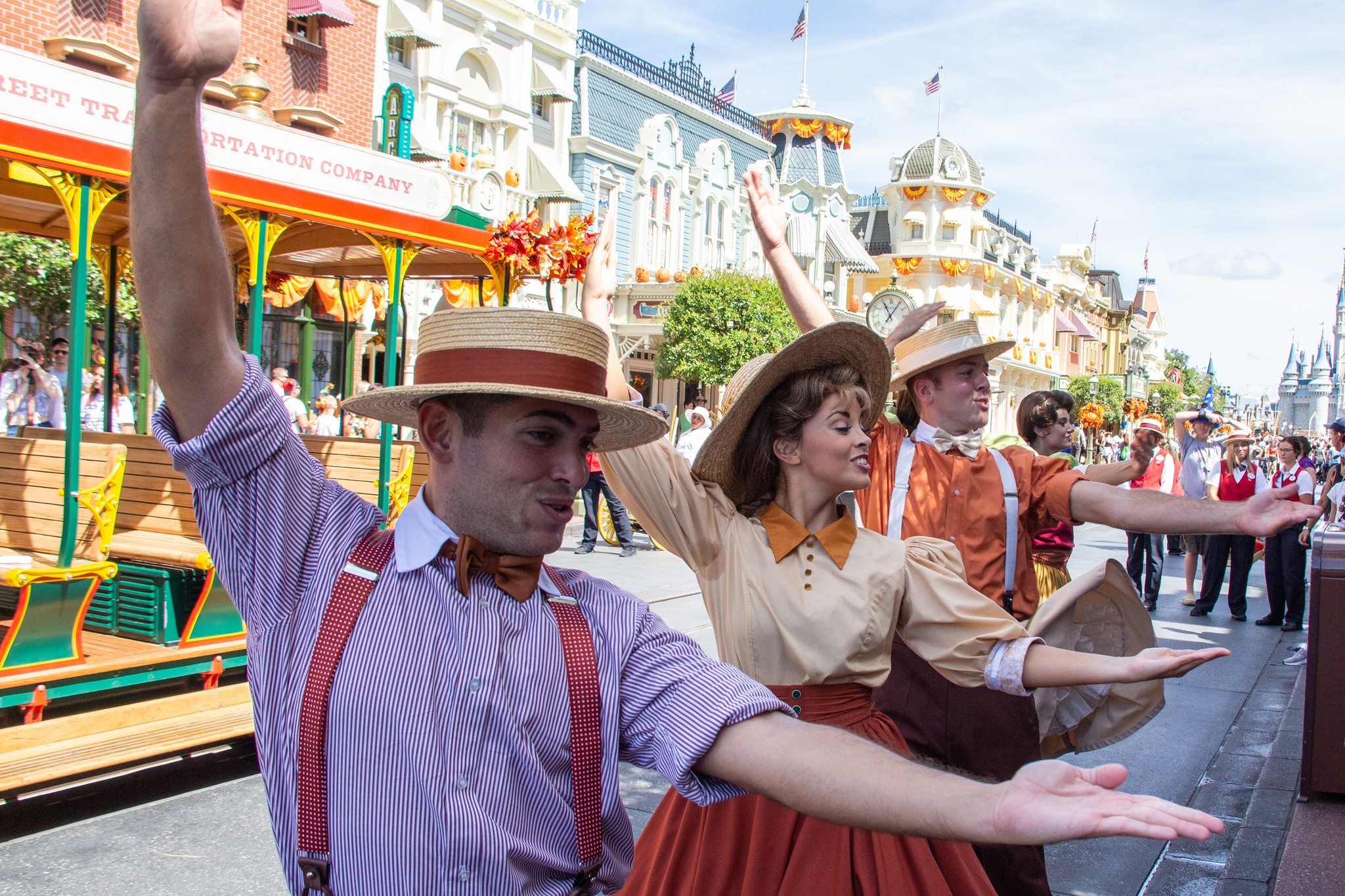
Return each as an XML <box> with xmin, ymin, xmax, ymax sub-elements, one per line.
<box><xmin>0</xmin><ymin>435</ymin><xmax>128</xmax><ymax>672</ymax></box>
<box><xmin>295</xmin><ymin>433</ymin><xmax>431</xmax><ymax>531</ymax></box>
<box><xmin>17</xmin><ymin>425</ymin><xmax>248</xmax><ymax>650</ymax></box>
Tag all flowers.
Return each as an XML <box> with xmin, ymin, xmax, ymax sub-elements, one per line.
<box><xmin>1217</xmin><ymin>425</ymin><xmax>1231</xmax><ymax>432</ymax></box>
<box><xmin>1183</xmin><ymin>421</ymin><xmax>1191</xmax><ymax>431</ymax></box>
<box><xmin>1124</xmin><ymin>397</ymin><xmax>1149</xmax><ymax>423</ymax></box>
<box><xmin>482</xmin><ymin>209</ymin><xmax>600</xmax><ymax>293</ymax></box>
<box><xmin>1145</xmin><ymin>413</ymin><xmax>1165</xmax><ymax>424</ymax></box>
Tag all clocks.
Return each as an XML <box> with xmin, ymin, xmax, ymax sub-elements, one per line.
<box><xmin>866</xmin><ymin>269</ymin><xmax>918</xmax><ymax>339</ymax></box>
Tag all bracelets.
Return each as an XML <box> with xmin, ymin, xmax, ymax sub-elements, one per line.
<box><xmin>1229</xmin><ymin>417</ymin><xmax>1233</xmax><ymax>424</ymax></box>
<box><xmin>1198</xmin><ymin>408</ymin><xmax>1206</xmax><ymax>418</ymax></box>
<box><xmin>1302</xmin><ymin>526</ymin><xmax>1311</xmax><ymax>532</ymax></box>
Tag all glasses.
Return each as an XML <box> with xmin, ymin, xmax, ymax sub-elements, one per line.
<box><xmin>282</xmin><ymin>376</ymin><xmax>289</xmax><ymax>379</ymax></box>
<box><xmin>1279</xmin><ymin>448</ymin><xmax>1296</xmax><ymax>454</ymax></box>
<box><xmin>53</xmin><ymin>350</ymin><xmax>68</xmax><ymax>355</ymax></box>
<box><xmin>652</xmin><ymin>409</ymin><xmax>658</xmax><ymax>412</ymax></box>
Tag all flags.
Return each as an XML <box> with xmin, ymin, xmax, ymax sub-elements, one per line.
<box><xmin>791</xmin><ymin>5</ymin><xmax>806</xmax><ymax>42</ymax></box>
<box><xmin>1144</xmin><ymin>245</ymin><xmax>1150</xmax><ymax>273</ymax></box>
<box><xmin>1091</xmin><ymin>220</ymin><xmax>1096</xmax><ymax>243</ymax></box>
<box><xmin>710</xmin><ymin>75</ymin><xmax>735</xmax><ymax>111</ymax></box>
<box><xmin>923</xmin><ymin>72</ymin><xmax>940</xmax><ymax>96</ymax></box>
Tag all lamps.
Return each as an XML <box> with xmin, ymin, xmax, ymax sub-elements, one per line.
<box><xmin>823</xmin><ymin>281</ymin><xmax>835</xmax><ymax>296</ymax></box>
<box><xmin>862</xmin><ymin>292</ymin><xmax>874</xmax><ymax>306</ymax></box>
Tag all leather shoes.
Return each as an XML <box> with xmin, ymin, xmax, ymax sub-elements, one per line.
<box><xmin>1143</xmin><ymin>599</ymin><xmax>1156</xmax><ymax>612</ymax></box>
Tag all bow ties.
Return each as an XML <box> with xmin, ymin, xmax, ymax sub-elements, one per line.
<box><xmin>438</xmin><ymin>533</ymin><xmax>545</xmax><ymax>604</ymax></box>
<box><xmin>931</xmin><ymin>426</ymin><xmax>982</xmax><ymax>461</ymax></box>
<box><xmin>1233</xmin><ymin>462</ymin><xmax>1246</xmax><ymax>472</ymax></box>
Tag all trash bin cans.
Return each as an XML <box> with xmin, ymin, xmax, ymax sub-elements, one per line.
<box><xmin>1296</xmin><ymin>520</ymin><xmax>1345</xmax><ymax>804</ymax></box>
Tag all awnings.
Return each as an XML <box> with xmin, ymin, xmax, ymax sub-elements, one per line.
<box><xmin>288</xmin><ymin>0</ymin><xmax>442</xmax><ymax>50</ymax></box>
<box><xmin>787</xmin><ymin>215</ymin><xmax>879</xmax><ymax>277</ymax></box>
<box><xmin>530</xmin><ymin>57</ymin><xmax>586</xmax><ymax>204</ymax></box>
<box><xmin>1055</xmin><ymin>310</ymin><xmax>1100</xmax><ymax>341</ymax></box>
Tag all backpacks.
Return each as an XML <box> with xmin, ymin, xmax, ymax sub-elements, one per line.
<box><xmin>1121</xmin><ymin>446</ymin><xmax>1129</xmax><ymax>460</ymax></box>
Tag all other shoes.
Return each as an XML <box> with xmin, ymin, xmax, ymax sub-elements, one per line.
<box><xmin>1255</xmin><ymin>615</ymin><xmax>1302</xmax><ymax>631</ymax></box>
<box><xmin>1189</xmin><ymin>607</ymin><xmax>1208</xmax><ymax>617</ymax></box>
<box><xmin>1168</xmin><ymin>549</ymin><xmax>1186</xmax><ymax>554</ymax></box>
<box><xmin>1231</xmin><ymin>613</ymin><xmax>1247</xmax><ymax>621</ymax></box>
<box><xmin>1182</xmin><ymin>593</ymin><xmax>1196</xmax><ymax>606</ymax></box>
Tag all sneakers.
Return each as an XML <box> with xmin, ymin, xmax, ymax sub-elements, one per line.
<box><xmin>575</xmin><ymin>545</ymin><xmax>593</xmax><ymax>554</ymax></box>
<box><xmin>1282</xmin><ymin>643</ymin><xmax>1307</xmax><ymax>666</ymax></box>
<box><xmin>620</xmin><ymin>546</ymin><xmax>637</xmax><ymax>557</ymax></box>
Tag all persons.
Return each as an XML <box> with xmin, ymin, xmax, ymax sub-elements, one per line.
<box><xmin>981</xmin><ymin>390</ymin><xmax>1155</xmax><ymax>611</ymax></box>
<box><xmin>1128</xmin><ymin>418</ymin><xmax>1175</xmax><ymax>610</ymax></box>
<box><xmin>1190</xmin><ymin>429</ymin><xmax>1269</xmax><ymax>621</ymax></box>
<box><xmin>270</xmin><ymin>367</ymin><xmax>399</xmax><ymax>441</ymax></box>
<box><xmin>1255</xmin><ymin>435</ymin><xmax>1316</xmax><ymax>632</ymax></box>
<box><xmin>1069</xmin><ymin>421</ymin><xmax>1130</xmax><ymax>466</ymax></box>
<box><xmin>1157</xmin><ymin>432</ymin><xmax>1187</xmax><ymax>555</ymax></box>
<box><xmin>1210</xmin><ymin>432</ymin><xmax>1340</xmax><ymax>484</ymax></box>
<box><xmin>579</xmin><ymin>207</ymin><xmax>1235</xmax><ymax>891</ymax></box>
<box><xmin>1175</xmin><ymin>386</ymin><xmax>1253</xmax><ymax>607</ymax></box>
<box><xmin>575</xmin><ymin>394</ymin><xmax>717</xmax><ymax>557</ymax></box>
<box><xmin>1282</xmin><ymin>417</ymin><xmax>1345</xmax><ymax>667</ymax></box>
<box><xmin>0</xmin><ymin>328</ymin><xmax>137</xmax><ymax>439</ymax></box>
<box><xmin>744</xmin><ymin>164</ymin><xmax>1322</xmax><ymax>895</ymax></box>
<box><xmin>131</xmin><ymin>2</ymin><xmax>1227</xmax><ymax>896</ymax></box>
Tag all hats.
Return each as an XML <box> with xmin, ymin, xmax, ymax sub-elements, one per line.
<box><xmin>1188</xmin><ymin>385</ymin><xmax>1223</xmax><ymax>429</ymax></box>
<box><xmin>339</xmin><ymin>306</ymin><xmax>672</xmax><ymax>454</ymax></box>
<box><xmin>1132</xmin><ymin>418</ymin><xmax>1165</xmax><ymax>439</ymax></box>
<box><xmin>889</xmin><ymin>319</ymin><xmax>1016</xmax><ymax>391</ymax></box>
<box><xmin>685</xmin><ymin>406</ymin><xmax>712</xmax><ymax>428</ymax></box>
<box><xmin>649</xmin><ymin>403</ymin><xmax>668</xmax><ymax>412</ymax></box>
<box><xmin>1333</xmin><ymin>446</ymin><xmax>1345</xmax><ymax>460</ymax></box>
<box><xmin>283</xmin><ymin>378</ymin><xmax>297</xmax><ymax>391</ymax></box>
<box><xmin>1221</xmin><ymin>430</ymin><xmax>1256</xmax><ymax>447</ymax></box>
<box><xmin>52</xmin><ymin>337</ymin><xmax>69</xmax><ymax>346</ymax></box>
<box><xmin>695</xmin><ymin>395</ymin><xmax>707</xmax><ymax>404</ymax></box>
<box><xmin>1323</xmin><ymin>417</ymin><xmax>1345</xmax><ymax>434</ymax></box>
<box><xmin>691</xmin><ymin>320</ymin><xmax>892</xmax><ymax>497</ymax></box>
